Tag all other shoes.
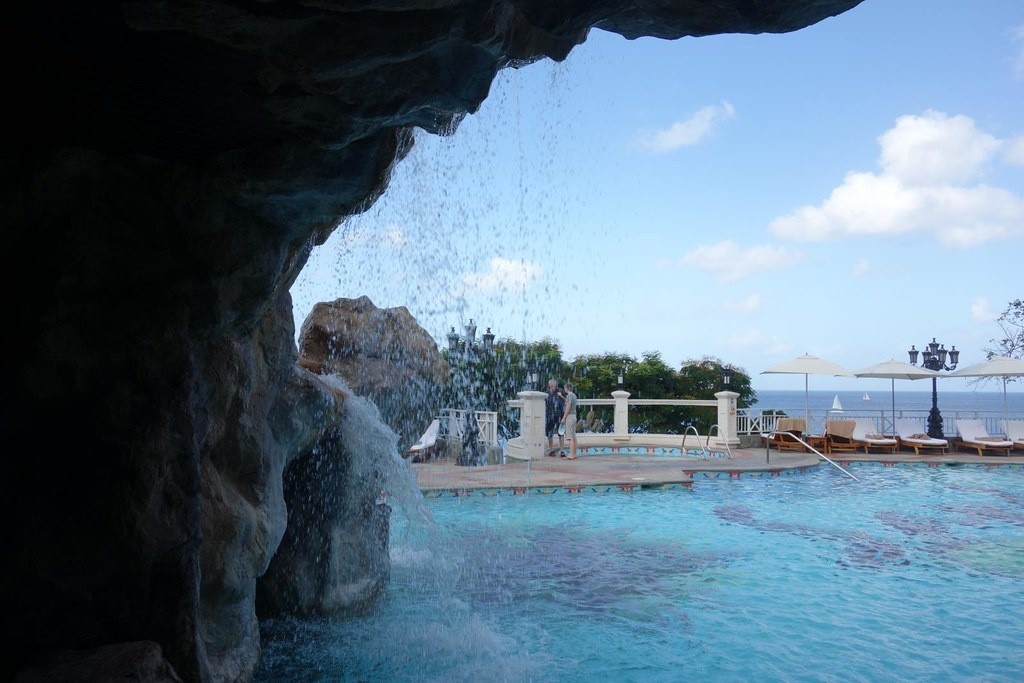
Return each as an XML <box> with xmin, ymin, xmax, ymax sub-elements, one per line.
<box><xmin>574</xmin><ymin>457</ymin><xmax>578</xmax><ymax>459</ymax></box>
<box><xmin>564</xmin><ymin>456</ymin><xmax>574</xmax><ymax>460</ymax></box>
<box><xmin>549</xmin><ymin>452</ymin><xmax>556</xmax><ymax>456</ymax></box>
<box><xmin>561</xmin><ymin>452</ymin><xmax>565</xmax><ymax>456</ymax></box>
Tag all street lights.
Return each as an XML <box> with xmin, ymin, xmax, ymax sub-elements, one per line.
<box><xmin>444</xmin><ymin>315</ymin><xmax>499</xmax><ymax>466</ymax></box>
<box><xmin>906</xmin><ymin>337</ymin><xmax>960</xmax><ymax>441</ymax></box>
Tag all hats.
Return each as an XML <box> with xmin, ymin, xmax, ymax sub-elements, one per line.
<box><xmin>558</xmin><ymin>424</ymin><xmax>566</xmax><ymax>436</ymax></box>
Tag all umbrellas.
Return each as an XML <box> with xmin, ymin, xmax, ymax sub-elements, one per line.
<box><xmin>946</xmin><ymin>355</ymin><xmax>1024</xmax><ymax>457</ymax></box>
<box><xmin>854</xmin><ymin>356</ymin><xmax>950</xmax><ymax>439</ymax></box>
<box><xmin>760</xmin><ymin>351</ymin><xmax>857</xmax><ymax>437</ymax></box>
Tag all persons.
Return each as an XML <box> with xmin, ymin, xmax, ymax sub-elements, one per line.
<box><xmin>557</xmin><ymin>384</ymin><xmax>578</xmax><ymax>460</ymax></box>
<box><xmin>544</xmin><ymin>380</ymin><xmax>566</xmax><ymax>457</ymax></box>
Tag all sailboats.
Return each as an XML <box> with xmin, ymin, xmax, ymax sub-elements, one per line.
<box><xmin>862</xmin><ymin>392</ymin><xmax>870</xmax><ymax>401</ymax></box>
<box><xmin>828</xmin><ymin>394</ymin><xmax>844</xmax><ymax>414</ymax></box>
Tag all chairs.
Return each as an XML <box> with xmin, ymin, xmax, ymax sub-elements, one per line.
<box><xmin>999</xmin><ymin>419</ymin><xmax>1024</xmax><ymax>450</ymax></box>
<box><xmin>407</xmin><ymin>418</ymin><xmax>439</xmax><ymax>462</ymax></box>
<box><xmin>761</xmin><ymin>417</ymin><xmax>808</xmax><ymax>454</ymax></box>
<box><xmin>895</xmin><ymin>421</ymin><xmax>948</xmax><ymax>456</ymax></box>
<box><xmin>808</xmin><ymin>418</ymin><xmax>858</xmax><ymax>455</ymax></box>
<box><xmin>856</xmin><ymin>421</ymin><xmax>897</xmax><ymax>456</ymax></box>
<box><xmin>952</xmin><ymin>420</ymin><xmax>1014</xmax><ymax>457</ymax></box>
